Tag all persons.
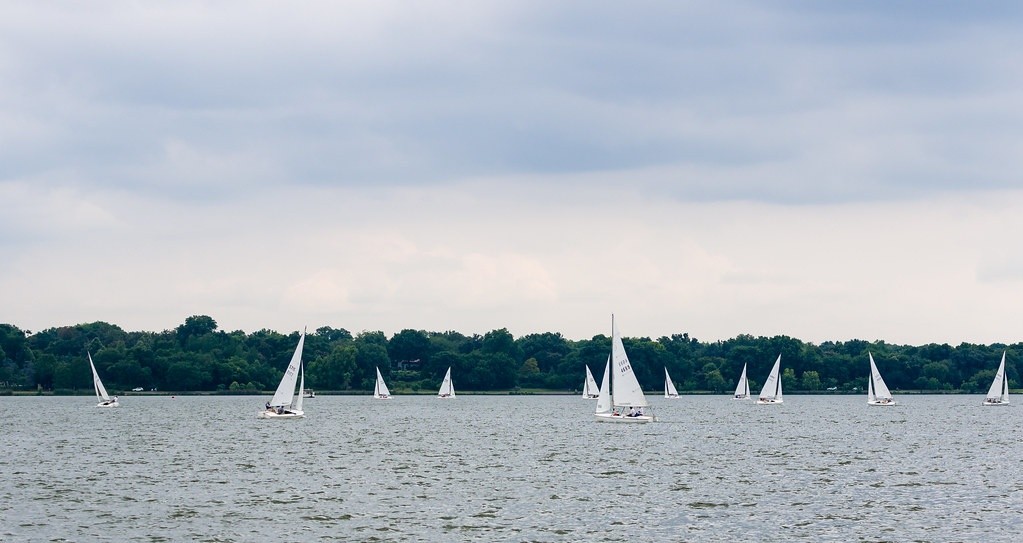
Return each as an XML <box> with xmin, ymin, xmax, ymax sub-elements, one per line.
<box><xmin>278</xmin><ymin>405</ymin><xmax>288</xmax><ymax>417</ymax></box>
<box><xmin>592</xmin><ymin>395</ymin><xmax>599</xmax><ymax>398</ymax></box>
<box><xmin>625</xmin><ymin>405</ymin><xmax>647</xmax><ymax>418</ymax></box>
<box><xmin>882</xmin><ymin>397</ymin><xmax>895</xmax><ymax>405</ymax></box>
<box><xmin>263</xmin><ymin>400</ymin><xmax>273</xmax><ymax>412</ymax></box>
<box><xmin>611</xmin><ymin>405</ymin><xmax>620</xmax><ymax>417</ymax></box>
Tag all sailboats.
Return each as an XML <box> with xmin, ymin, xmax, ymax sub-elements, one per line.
<box><xmin>258</xmin><ymin>328</ymin><xmax>306</xmax><ymax>419</ymax></box>
<box><xmin>754</xmin><ymin>353</ymin><xmax>784</xmax><ymax>404</ymax></box>
<box><xmin>867</xmin><ymin>351</ymin><xmax>899</xmax><ymax>407</ymax></box>
<box><xmin>582</xmin><ymin>313</ymin><xmax>662</xmax><ymax>424</ymax></box>
<box><xmin>437</xmin><ymin>367</ymin><xmax>456</xmax><ymax>399</ymax></box>
<box><xmin>664</xmin><ymin>367</ymin><xmax>682</xmax><ymax>399</ymax></box>
<box><xmin>731</xmin><ymin>362</ymin><xmax>751</xmax><ymax>400</ymax></box>
<box><xmin>88</xmin><ymin>351</ymin><xmax>120</xmax><ymax>407</ymax></box>
<box><xmin>371</xmin><ymin>367</ymin><xmax>394</xmax><ymax>399</ymax></box>
<box><xmin>982</xmin><ymin>350</ymin><xmax>1011</xmax><ymax>405</ymax></box>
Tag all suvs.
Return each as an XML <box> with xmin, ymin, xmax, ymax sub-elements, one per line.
<box><xmin>133</xmin><ymin>387</ymin><xmax>144</xmax><ymax>392</ymax></box>
<box><xmin>828</xmin><ymin>386</ymin><xmax>838</xmax><ymax>391</ymax></box>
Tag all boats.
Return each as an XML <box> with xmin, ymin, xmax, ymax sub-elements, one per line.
<box><xmin>304</xmin><ymin>389</ymin><xmax>315</xmax><ymax>398</ymax></box>
